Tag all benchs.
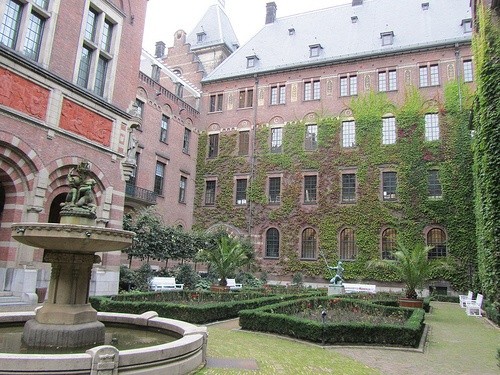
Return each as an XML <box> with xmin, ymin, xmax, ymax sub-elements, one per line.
<box><xmin>458</xmin><ymin>290</ymin><xmax>474</xmax><ymax>309</ymax></box>
<box><xmin>217</xmin><ymin>278</ymin><xmax>243</xmax><ymax>291</ymax></box>
<box><xmin>146</xmin><ymin>276</ymin><xmax>185</xmax><ymax>293</ymax></box>
<box><xmin>465</xmin><ymin>293</ymin><xmax>484</xmax><ymax>317</ymax></box>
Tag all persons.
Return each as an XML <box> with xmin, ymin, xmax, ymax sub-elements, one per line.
<box><xmin>65</xmin><ymin>160</ymin><xmax>97</xmax><ymax>215</ymax></box>
<box><xmin>327</xmin><ymin>261</ymin><xmax>345</xmax><ymax>285</ymax></box>
